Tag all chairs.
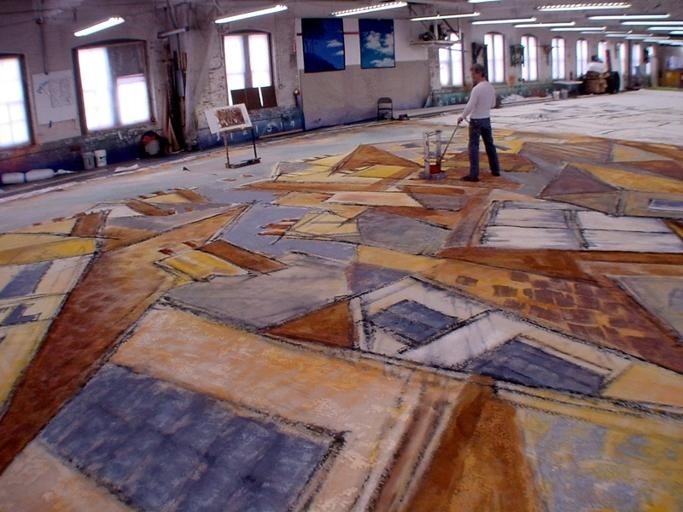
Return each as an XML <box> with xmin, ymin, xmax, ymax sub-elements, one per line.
<box><xmin>377</xmin><ymin>97</ymin><xmax>393</xmax><ymax>121</ymax></box>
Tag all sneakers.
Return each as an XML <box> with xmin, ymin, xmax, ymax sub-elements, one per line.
<box><xmin>463</xmin><ymin>171</ymin><xmax>500</xmax><ymax>182</ymax></box>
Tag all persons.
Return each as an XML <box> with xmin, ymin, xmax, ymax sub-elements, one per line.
<box><xmin>457</xmin><ymin>64</ymin><xmax>500</xmax><ymax>181</ymax></box>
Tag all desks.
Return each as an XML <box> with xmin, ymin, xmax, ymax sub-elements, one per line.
<box><xmin>553</xmin><ymin>80</ymin><xmax>584</xmax><ymax>98</ymax></box>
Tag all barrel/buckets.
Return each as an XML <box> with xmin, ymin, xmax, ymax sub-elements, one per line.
<box><xmin>94</xmin><ymin>149</ymin><xmax>107</xmax><ymax>166</ymax></box>
<box><xmin>81</xmin><ymin>152</ymin><xmax>95</xmax><ymax>169</ymax></box>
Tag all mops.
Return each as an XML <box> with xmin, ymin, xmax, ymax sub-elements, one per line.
<box><xmin>417</xmin><ymin>119</ymin><xmax>462</xmax><ymax>179</ymax></box>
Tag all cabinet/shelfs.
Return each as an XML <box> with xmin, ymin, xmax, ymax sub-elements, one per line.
<box><xmin>407</xmin><ymin>2</ymin><xmax>463</xmax><ymax>48</ymax></box>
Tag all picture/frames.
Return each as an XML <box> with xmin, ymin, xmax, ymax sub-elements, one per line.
<box><xmin>358</xmin><ymin>17</ymin><xmax>396</xmax><ymax>70</ymax></box>
<box><xmin>301</xmin><ymin>17</ymin><xmax>345</xmax><ymax>73</ymax></box>
<box><xmin>472</xmin><ymin>41</ymin><xmax>488</xmax><ymax>86</ymax></box>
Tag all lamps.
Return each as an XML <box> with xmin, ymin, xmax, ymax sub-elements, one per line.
<box><xmin>328</xmin><ymin>1</ymin><xmax>408</xmax><ymax>18</ymax></box>
<box><xmin>72</xmin><ymin>15</ymin><xmax>125</xmax><ymax>38</ymax></box>
<box><xmin>408</xmin><ymin>12</ymin><xmax>481</xmax><ymax>23</ymax></box>
<box><xmin>211</xmin><ymin>4</ymin><xmax>288</xmax><ymax>24</ymax></box>
<box><xmin>471</xmin><ymin>3</ymin><xmax>683</xmax><ymax>47</ymax></box>
